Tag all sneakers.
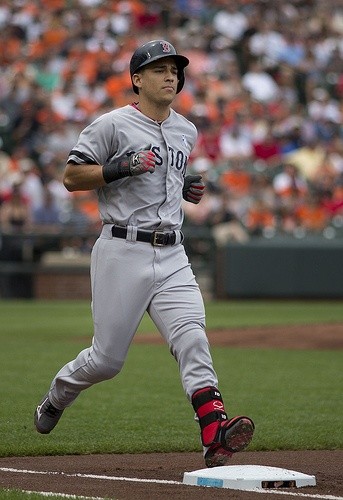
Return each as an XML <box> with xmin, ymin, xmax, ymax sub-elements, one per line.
<box><xmin>33</xmin><ymin>391</ymin><xmax>64</xmax><ymax>435</ymax></box>
<box><xmin>204</xmin><ymin>416</ymin><xmax>255</xmax><ymax>468</ymax></box>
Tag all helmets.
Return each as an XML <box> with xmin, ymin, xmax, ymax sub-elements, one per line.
<box><xmin>129</xmin><ymin>39</ymin><xmax>190</xmax><ymax>95</ymax></box>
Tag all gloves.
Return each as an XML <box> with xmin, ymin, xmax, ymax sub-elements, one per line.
<box><xmin>182</xmin><ymin>175</ymin><xmax>206</xmax><ymax>204</ymax></box>
<box><xmin>102</xmin><ymin>150</ymin><xmax>157</xmax><ymax>184</ymax></box>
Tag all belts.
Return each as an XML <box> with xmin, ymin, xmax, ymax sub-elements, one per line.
<box><xmin>111</xmin><ymin>226</ymin><xmax>184</xmax><ymax>247</ymax></box>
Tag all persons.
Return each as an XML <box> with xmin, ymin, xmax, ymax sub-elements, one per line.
<box><xmin>0</xmin><ymin>0</ymin><xmax>343</xmax><ymax>262</ymax></box>
<box><xmin>31</xmin><ymin>40</ymin><xmax>255</xmax><ymax>469</ymax></box>
